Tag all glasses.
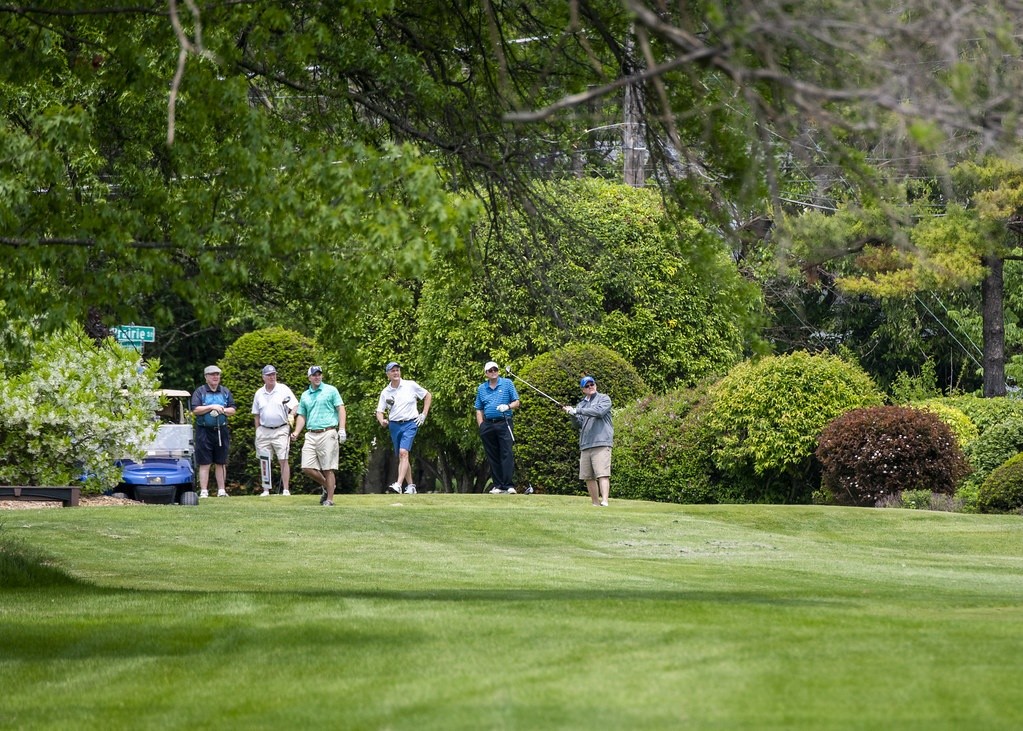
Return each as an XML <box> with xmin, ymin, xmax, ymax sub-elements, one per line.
<box><xmin>584</xmin><ymin>383</ymin><xmax>594</xmax><ymax>388</ymax></box>
<box><xmin>488</xmin><ymin>370</ymin><xmax>497</xmax><ymax>372</ymax></box>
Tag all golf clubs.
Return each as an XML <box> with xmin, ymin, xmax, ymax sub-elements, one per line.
<box><xmin>282</xmin><ymin>395</ymin><xmax>295</xmax><ymax>436</ymax></box>
<box><xmin>502</xmin><ymin>412</ymin><xmax>534</xmax><ymax>494</ymax></box>
<box><xmin>505</xmin><ymin>365</ymin><xmax>570</xmax><ymax>412</ymax></box>
<box><xmin>386</xmin><ymin>399</ymin><xmax>394</xmax><ymax>421</ymax></box>
<box><xmin>216</xmin><ymin>416</ymin><xmax>227</xmax><ymax>497</ymax></box>
<box><xmin>273</xmin><ymin>426</ymin><xmax>291</xmax><ymax>496</ymax></box>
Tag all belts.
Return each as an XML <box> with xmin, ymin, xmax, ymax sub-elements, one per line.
<box><xmin>389</xmin><ymin>418</ymin><xmax>416</xmax><ymax>423</ymax></box>
<box><xmin>260</xmin><ymin>423</ymin><xmax>287</xmax><ymax>429</ymax></box>
<box><xmin>307</xmin><ymin>427</ymin><xmax>334</xmax><ymax>433</ymax></box>
<box><xmin>484</xmin><ymin>418</ymin><xmax>512</xmax><ymax>424</ymax></box>
<box><xmin>197</xmin><ymin>425</ymin><xmax>223</xmax><ymax>431</ymax></box>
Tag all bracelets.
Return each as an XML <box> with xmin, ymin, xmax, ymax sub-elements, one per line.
<box><xmin>507</xmin><ymin>404</ymin><xmax>510</xmax><ymax>410</ymax></box>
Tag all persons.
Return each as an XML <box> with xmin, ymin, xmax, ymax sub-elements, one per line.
<box><xmin>290</xmin><ymin>366</ymin><xmax>347</xmax><ymax>506</ymax></box>
<box><xmin>563</xmin><ymin>377</ymin><xmax>615</xmax><ymax>507</ymax></box>
<box><xmin>376</xmin><ymin>362</ymin><xmax>431</xmax><ymax>494</ymax></box>
<box><xmin>191</xmin><ymin>365</ymin><xmax>238</xmax><ymax>498</ymax></box>
<box><xmin>252</xmin><ymin>365</ymin><xmax>299</xmax><ymax>497</ymax></box>
<box><xmin>474</xmin><ymin>362</ymin><xmax>520</xmax><ymax>494</ymax></box>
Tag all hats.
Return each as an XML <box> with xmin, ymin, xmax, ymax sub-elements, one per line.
<box><xmin>485</xmin><ymin>362</ymin><xmax>498</xmax><ymax>371</ymax></box>
<box><xmin>262</xmin><ymin>365</ymin><xmax>278</xmax><ymax>375</ymax></box>
<box><xmin>386</xmin><ymin>362</ymin><xmax>400</xmax><ymax>372</ymax></box>
<box><xmin>308</xmin><ymin>366</ymin><xmax>323</xmax><ymax>377</ymax></box>
<box><xmin>581</xmin><ymin>377</ymin><xmax>595</xmax><ymax>387</ymax></box>
<box><xmin>204</xmin><ymin>365</ymin><xmax>221</xmax><ymax>374</ymax></box>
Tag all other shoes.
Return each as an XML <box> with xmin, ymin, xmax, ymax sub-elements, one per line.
<box><xmin>592</xmin><ymin>501</ymin><xmax>608</xmax><ymax>506</ymax></box>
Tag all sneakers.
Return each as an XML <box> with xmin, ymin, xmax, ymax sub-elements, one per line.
<box><xmin>489</xmin><ymin>487</ymin><xmax>517</xmax><ymax>494</ymax></box>
<box><xmin>200</xmin><ymin>492</ymin><xmax>209</xmax><ymax>498</ymax></box>
<box><xmin>217</xmin><ymin>491</ymin><xmax>228</xmax><ymax>497</ymax></box>
<box><xmin>389</xmin><ymin>483</ymin><xmax>402</xmax><ymax>494</ymax></box>
<box><xmin>323</xmin><ymin>500</ymin><xmax>333</xmax><ymax>507</ymax></box>
<box><xmin>283</xmin><ymin>490</ymin><xmax>290</xmax><ymax>496</ymax></box>
<box><xmin>320</xmin><ymin>485</ymin><xmax>336</xmax><ymax>503</ymax></box>
<box><xmin>260</xmin><ymin>491</ymin><xmax>269</xmax><ymax>496</ymax></box>
<box><xmin>404</xmin><ymin>485</ymin><xmax>417</xmax><ymax>494</ymax></box>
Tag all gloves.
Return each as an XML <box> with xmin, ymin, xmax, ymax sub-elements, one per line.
<box><xmin>210</xmin><ymin>409</ymin><xmax>219</xmax><ymax>417</ymax></box>
<box><xmin>288</xmin><ymin>414</ymin><xmax>294</xmax><ymax>428</ymax></box>
<box><xmin>566</xmin><ymin>406</ymin><xmax>576</xmax><ymax>416</ymax></box>
<box><xmin>338</xmin><ymin>428</ymin><xmax>346</xmax><ymax>444</ymax></box>
<box><xmin>497</xmin><ymin>404</ymin><xmax>509</xmax><ymax>412</ymax></box>
<box><xmin>413</xmin><ymin>414</ymin><xmax>426</xmax><ymax>427</ymax></box>
